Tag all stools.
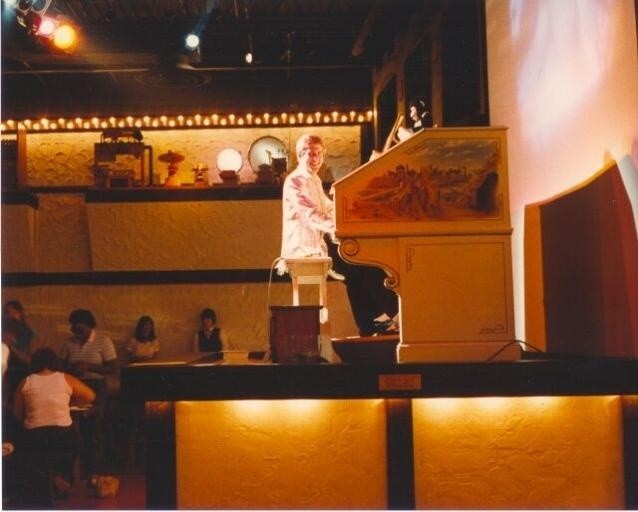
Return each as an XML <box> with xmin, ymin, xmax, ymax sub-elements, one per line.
<box><xmin>283</xmin><ymin>257</ymin><xmax>333</xmax><ymax>361</ymax></box>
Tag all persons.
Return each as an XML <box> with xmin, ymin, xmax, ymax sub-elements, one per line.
<box><xmin>279</xmin><ymin>135</ymin><xmax>400</xmax><ymax>337</ymax></box>
<box><xmin>193</xmin><ymin>308</ymin><xmax>228</xmax><ymax>353</ymax></box>
<box><xmin>13</xmin><ymin>347</ymin><xmax>96</xmax><ymax>496</ymax></box>
<box><xmin>124</xmin><ymin>316</ymin><xmax>159</xmax><ymax>360</ymax></box>
<box><xmin>2</xmin><ymin>299</ymin><xmax>34</xmax><ymax>378</ymax></box>
<box><xmin>59</xmin><ymin>309</ymin><xmax>117</xmax><ymax>406</ymax></box>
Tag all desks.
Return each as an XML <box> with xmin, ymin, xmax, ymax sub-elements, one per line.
<box><xmin>66</xmin><ymin>404</ymin><xmax>91</xmax><ymax>480</ymax></box>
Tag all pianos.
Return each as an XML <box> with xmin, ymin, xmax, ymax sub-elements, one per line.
<box><xmin>328</xmin><ymin>126</ymin><xmax>523</xmax><ymax>365</ymax></box>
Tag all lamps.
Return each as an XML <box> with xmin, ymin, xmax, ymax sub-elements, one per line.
<box><xmin>13</xmin><ymin>0</ymin><xmax>80</xmax><ymax>55</ymax></box>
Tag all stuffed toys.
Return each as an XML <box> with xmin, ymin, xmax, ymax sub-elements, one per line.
<box><xmin>397</xmin><ymin>96</ymin><xmax>434</xmax><ymax>139</ymax></box>
